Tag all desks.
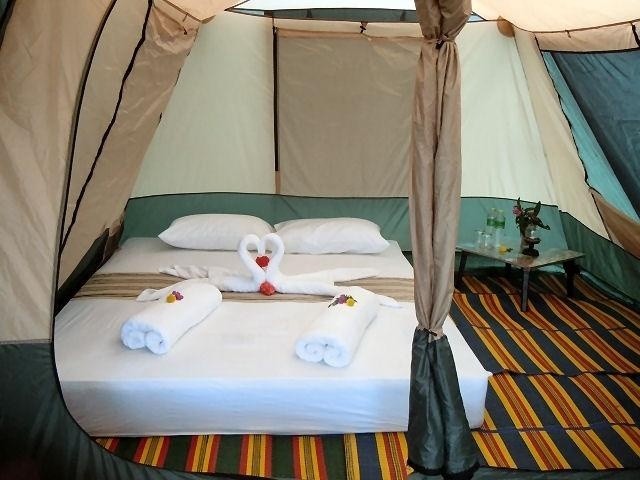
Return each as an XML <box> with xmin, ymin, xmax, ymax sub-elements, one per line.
<box><xmin>453</xmin><ymin>237</ymin><xmax>586</xmax><ymax>312</ymax></box>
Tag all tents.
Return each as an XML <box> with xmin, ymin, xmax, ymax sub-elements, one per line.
<box><xmin>0</xmin><ymin>0</ymin><xmax>640</xmax><ymax>480</ymax></box>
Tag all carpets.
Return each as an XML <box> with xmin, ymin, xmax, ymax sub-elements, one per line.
<box><xmin>94</xmin><ymin>269</ymin><xmax>640</xmax><ymax>478</ymax></box>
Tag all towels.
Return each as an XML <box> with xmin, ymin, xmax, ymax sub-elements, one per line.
<box><xmin>120</xmin><ymin>235</ymin><xmax>404</xmax><ymax>369</ymax></box>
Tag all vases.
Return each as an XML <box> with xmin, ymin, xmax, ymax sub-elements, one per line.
<box><xmin>520</xmin><ymin>211</ymin><xmax>536</xmax><ymax>250</ymax></box>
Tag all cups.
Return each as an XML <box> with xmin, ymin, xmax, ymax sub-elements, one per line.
<box><xmin>473</xmin><ymin>229</ymin><xmax>494</xmax><ymax>253</ymax></box>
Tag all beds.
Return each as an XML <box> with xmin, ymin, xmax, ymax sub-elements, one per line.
<box><xmin>52</xmin><ymin>234</ymin><xmax>492</xmax><ymax>436</ymax></box>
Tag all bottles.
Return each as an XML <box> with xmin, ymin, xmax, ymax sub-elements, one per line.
<box><xmin>486</xmin><ymin>207</ymin><xmax>506</xmax><ymax>250</ymax></box>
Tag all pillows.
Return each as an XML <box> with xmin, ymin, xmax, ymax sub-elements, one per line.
<box><xmin>158</xmin><ymin>213</ymin><xmax>392</xmax><ymax>255</ymax></box>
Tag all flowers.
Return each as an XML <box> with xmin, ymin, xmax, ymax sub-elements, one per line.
<box><xmin>512</xmin><ymin>195</ymin><xmax>551</xmax><ymax>239</ymax></box>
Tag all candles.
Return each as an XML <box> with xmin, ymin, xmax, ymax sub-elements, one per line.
<box><xmin>529</xmin><ymin>229</ymin><xmax>535</xmax><ymax>239</ymax></box>
